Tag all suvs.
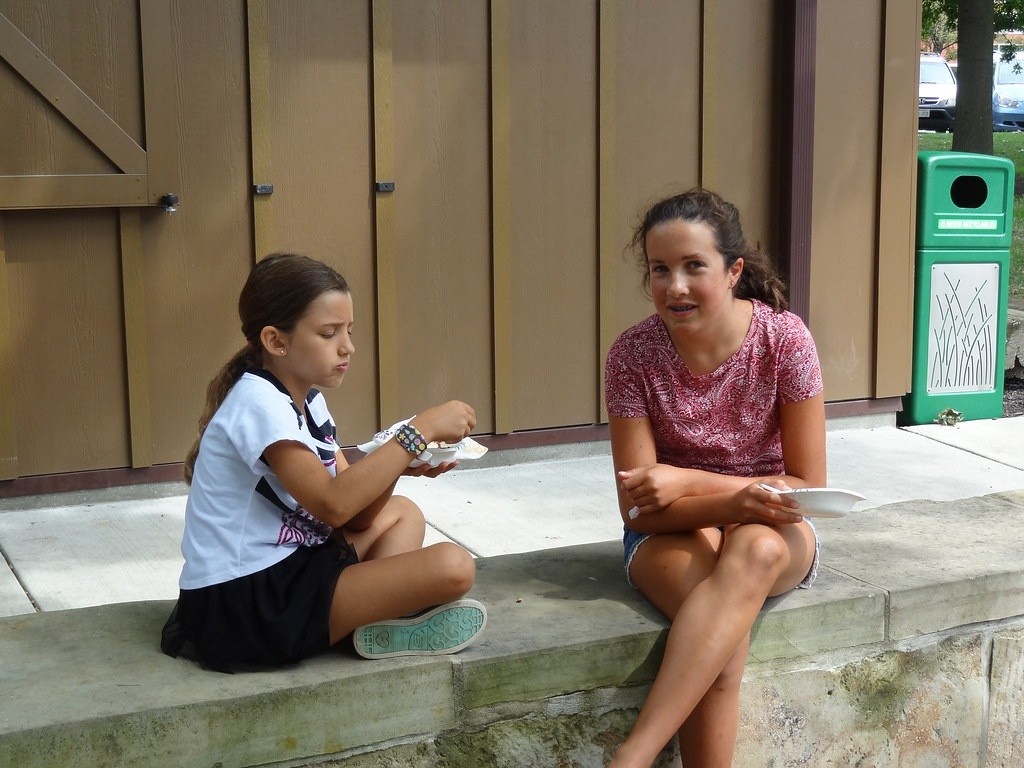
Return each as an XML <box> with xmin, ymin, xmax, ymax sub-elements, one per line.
<box><xmin>919</xmin><ymin>52</ymin><xmax>958</xmax><ymax>134</ymax></box>
<box><xmin>992</xmin><ymin>51</ymin><xmax>1024</xmax><ymax>132</ymax></box>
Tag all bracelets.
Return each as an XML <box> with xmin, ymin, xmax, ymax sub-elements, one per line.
<box><xmin>395</xmin><ymin>424</ymin><xmax>432</xmax><ymax>462</ymax></box>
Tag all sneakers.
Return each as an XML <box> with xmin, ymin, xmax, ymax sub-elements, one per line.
<box><xmin>354</xmin><ymin>599</ymin><xmax>490</xmax><ymax>660</ymax></box>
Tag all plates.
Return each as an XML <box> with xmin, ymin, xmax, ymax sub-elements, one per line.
<box><xmin>775</xmin><ymin>489</ymin><xmax>867</xmax><ymax>517</ymax></box>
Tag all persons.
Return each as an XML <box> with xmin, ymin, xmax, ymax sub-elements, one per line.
<box><xmin>159</xmin><ymin>251</ymin><xmax>488</xmax><ymax>673</ymax></box>
<box><xmin>604</xmin><ymin>191</ymin><xmax>826</xmax><ymax>768</ymax></box>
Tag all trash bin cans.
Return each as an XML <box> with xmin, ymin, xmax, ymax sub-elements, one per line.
<box><xmin>908</xmin><ymin>149</ymin><xmax>1015</xmax><ymax>424</ymax></box>
